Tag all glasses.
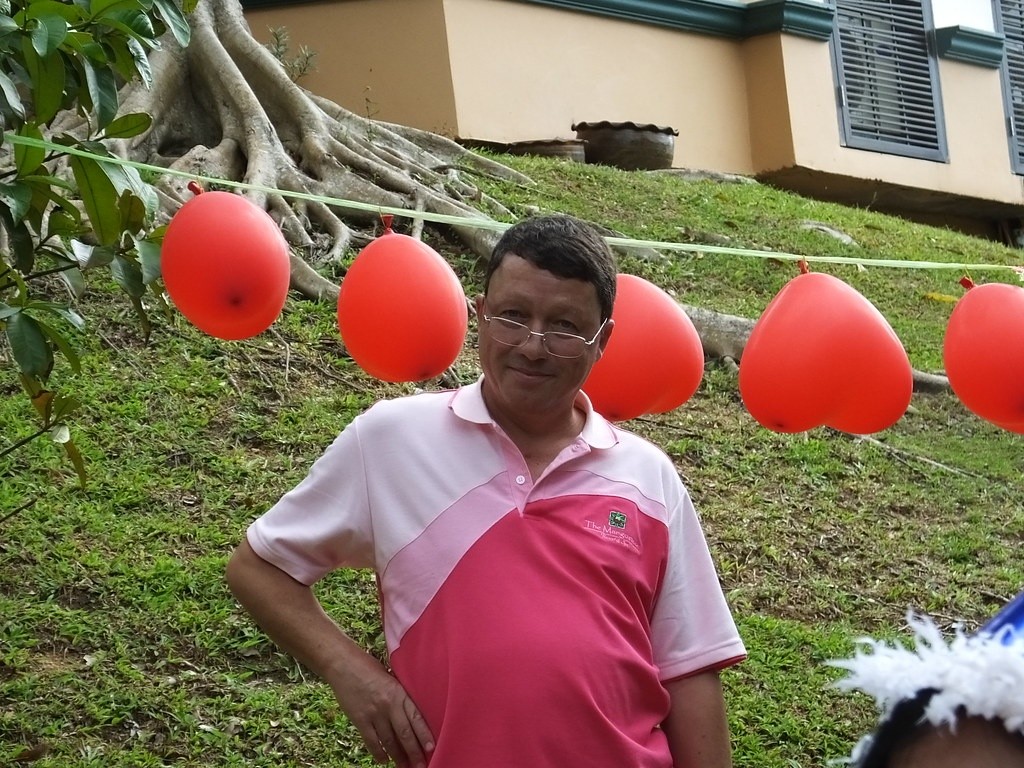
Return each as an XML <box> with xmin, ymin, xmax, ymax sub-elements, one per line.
<box><xmin>483</xmin><ymin>297</ymin><xmax>607</xmax><ymax>359</ymax></box>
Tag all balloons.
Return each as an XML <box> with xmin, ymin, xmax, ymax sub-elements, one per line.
<box><xmin>943</xmin><ymin>276</ymin><xmax>1024</xmax><ymax>434</ymax></box>
<box><xmin>582</xmin><ymin>275</ymin><xmax>704</xmax><ymax>421</ymax></box>
<box><xmin>338</xmin><ymin>217</ymin><xmax>467</xmax><ymax>382</ymax></box>
<box><xmin>162</xmin><ymin>182</ymin><xmax>290</xmax><ymax>340</ymax></box>
<box><xmin>741</xmin><ymin>263</ymin><xmax>914</xmax><ymax>433</ymax></box>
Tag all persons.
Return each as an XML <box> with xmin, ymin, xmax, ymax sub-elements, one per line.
<box><xmin>227</xmin><ymin>216</ymin><xmax>748</xmax><ymax>768</ymax></box>
<box><xmin>835</xmin><ymin>617</ymin><xmax>1024</xmax><ymax>768</ymax></box>
